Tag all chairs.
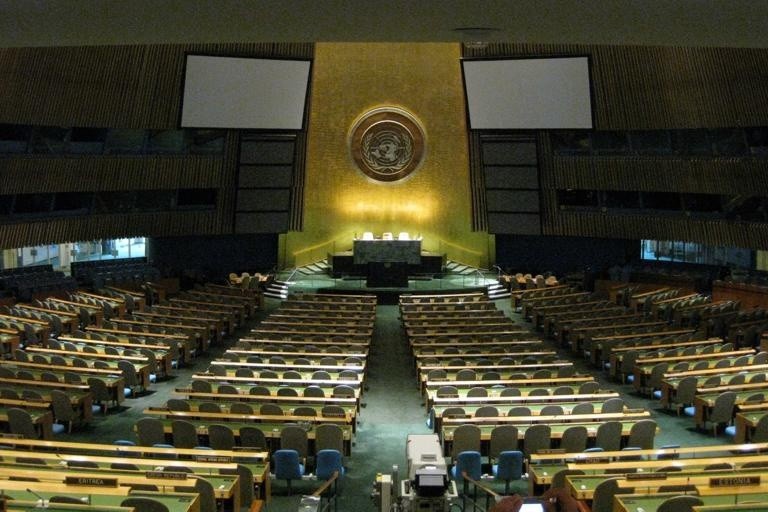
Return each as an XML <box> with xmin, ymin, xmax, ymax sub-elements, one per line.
<box><xmin>0</xmin><ymin>272</ymin><xmax>378</xmax><ymax>512</ymax></box>
<box><xmin>397</xmin><ymin>273</ymin><xmax>768</xmax><ymax>512</ymax></box>
